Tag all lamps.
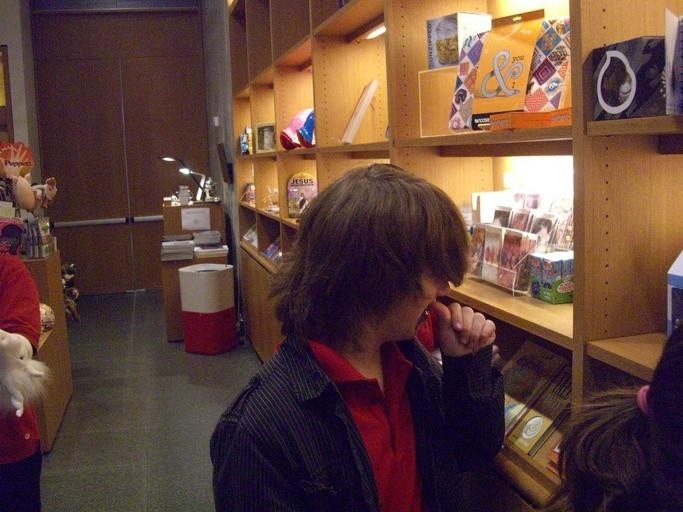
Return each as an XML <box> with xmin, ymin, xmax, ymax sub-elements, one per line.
<box><xmin>178</xmin><ymin>168</ymin><xmax>206</xmax><ymax>202</ymax></box>
<box><xmin>157</xmin><ymin>155</ymin><xmax>212</xmax><ymax>204</ymax></box>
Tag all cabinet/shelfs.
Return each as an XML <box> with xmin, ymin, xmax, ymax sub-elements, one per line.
<box><xmin>19</xmin><ymin>251</ymin><xmax>74</xmax><ymax>454</ymax></box>
<box><xmin>225</xmin><ymin>0</ymin><xmax>683</xmax><ymax>512</ymax></box>
<box><xmin>161</xmin><ymin>203</ymin><xmax>228</xmax><ymax>342</ymax></box>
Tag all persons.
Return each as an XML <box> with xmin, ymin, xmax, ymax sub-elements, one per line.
<box><xmin>0</xmin><ymin>248</ymin><xmax>44</xmax><ymax>512</ymax></box>
<box><xmin>555</xmin><ymin>318</ymin><xmax>683</xmax><ymax>511</ymax></box>
<box><xmin>207</xmin><ymin>161</ymin><xmax>509</xmax><ymax>512</ymax></box>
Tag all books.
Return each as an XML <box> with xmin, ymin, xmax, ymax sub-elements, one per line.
<box><xmin>458</xmin><ymin>186</ymin><xmax>572</xmax><ymax>480</ymax></box>
<box><xmin>159</xmin><ymin>227</ymin><xmax>230</xmax><ymax>263</ymax></box>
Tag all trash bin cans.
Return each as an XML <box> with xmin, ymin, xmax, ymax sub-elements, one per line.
<box><xmin>178</xmin><ymin>263</ymin><xmax>239</xmax><ymax>355</ymax></box>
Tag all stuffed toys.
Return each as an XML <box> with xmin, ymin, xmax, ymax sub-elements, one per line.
<box><xmin>0</xmin><ymin>329</ymin><xmax>50</xmax><ymax>420</ymax></box>
<box><xmin>0</xmin><ymin>168</ymin><xmax>83</xmax><ymax>336</ymax></box>
